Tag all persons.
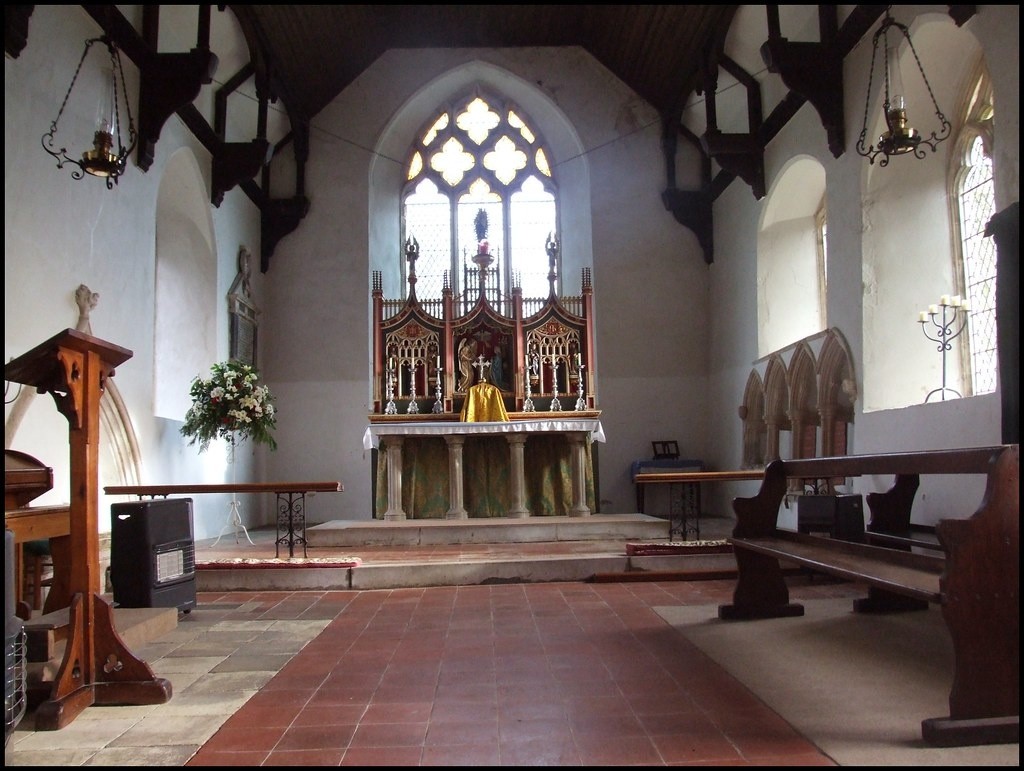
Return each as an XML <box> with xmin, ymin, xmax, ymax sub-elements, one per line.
<box><xmin>457</xmin><ymin>338</ymin><xmax>478</xmax><ymax>392</ymax></box>
<box><xmin>489</xmin><ymin>345</ymin><xmax>503</xmax><ymax>390</ymax></box>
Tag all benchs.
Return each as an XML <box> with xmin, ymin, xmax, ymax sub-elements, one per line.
<box><xmin>718</xmin><ymin>443</ymin><xmax>1020</xmax><ymax>749</ymax></box>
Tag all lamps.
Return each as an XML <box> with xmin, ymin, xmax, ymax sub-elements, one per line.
<box><xmin>856</xmin><ymin>5</ymin><xmax>952</xmax><ymax>168</ymax></box>
<box><xmin>42</xmin><ymin>34</ymin><xmax>139</xmax><ymax>188</ymax></box>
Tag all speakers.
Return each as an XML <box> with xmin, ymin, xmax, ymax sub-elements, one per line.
<box><xmin>110</xmin><ymin>498</ymin><xmax>196</xmax><ymax>612</ymax></box>
<box><xmin>797</xmin><ymin>493</ymin><xmax>865</xmax><ymax>543</ymax></box>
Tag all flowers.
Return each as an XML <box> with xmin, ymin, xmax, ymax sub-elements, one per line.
<box><xmin>179</xmin><ymin>358</ymin><xmax>278</xmax><ymax>457</ymax></box>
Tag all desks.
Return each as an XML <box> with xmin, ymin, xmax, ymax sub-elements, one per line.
<box><xmin>634</xmin><ymin>471</ymin><xmax>765</xmax><ymax>542</ymax></box>
<box><xmin>4</xmin><ymin>504</ymin><xmax>70</xmax><ymax>620</ymax></box>
<box><xmin>777</xmin><ymin>492</ymin><xmax>865</xmax><ymax>584</ymax></box>
<box><xmin>104</xmin><ymin>481</ymin><xmax>345</xmax><ymax>558</ymax></box>
<box><xmin>630</xmin><ymin>459</ymin><xmax>706</xmax><ymax>519</ymax></box>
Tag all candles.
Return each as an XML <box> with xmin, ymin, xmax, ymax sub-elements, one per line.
<box><xmin>551</xmin><ymin>354</ymin><xmax>556</xmax><ymax>366</ymax></box>
<box><xmin>389</xmin><ymin>357</ymin><xmax>393</xmax><ymax>369</ymax></box>
<box><xmin>577</xmin><ymin>353</ymin><xmax>581</xmax><ymax>367</ymax></box>
<box><xmin>524</xmin><ymin>355</ymin><xmax>529</xmax><ymax>367</ymax></box>
<box><xmin>961</xmin><ymin>299</ymin><xmax>971</xmax><ymax>311</ymax></box>
<box><xmin>436</xmin><ymin>355</ymin><xmax>440</xmax><ymax>368</ymax></box>
<box><xmin>952</xmin><ymin>295</ymin><xmax>961</xmax><ymax>306</ymax></box>
<box><xmin>411</xmin><ymin>357</ymin><xmax>414</xmax><ymax>369</ymax></box>
<box><xmin>929</xmin><ymin>304</ymin><xmax>938</xmax><ymax>313</ymax></box>
<box><xmin>919</xmin><ymin>311</ymin><xmax>928</xmax><ymax>321</ymax></box>
<box><xmin>941</xmin><ymin>295</ymin><xmax>950</xmax><ymax>305</ymax></box>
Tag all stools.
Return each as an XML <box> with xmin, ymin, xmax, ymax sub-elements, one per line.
<box><xmin>16</xmin><ymin>606</ymin><xmax>70</xmax><ymax>663</ymax></box>
